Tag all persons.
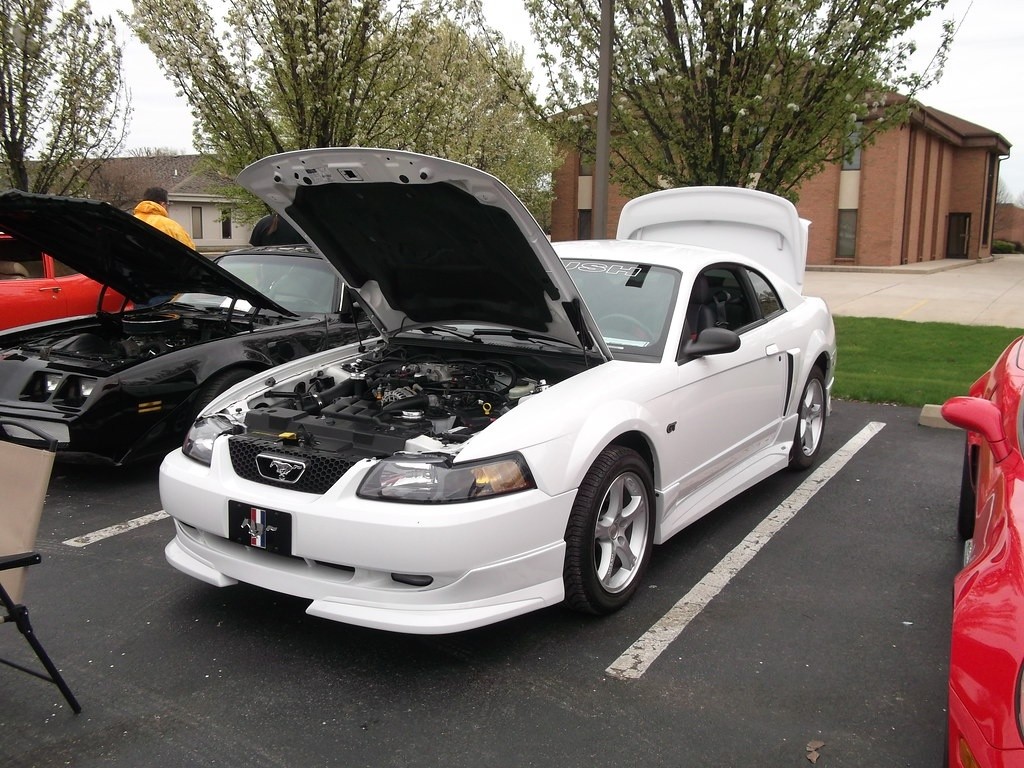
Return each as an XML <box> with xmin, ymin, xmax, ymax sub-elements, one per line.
<box><xmin>250</xmin><ymin>211</ymin><xmax>306</xmax><ymax>288</ymax></box>
<box><xmin>133</xmin><ymin>188</ymin><xmax>195</xmax><ymax>308</ymax></box>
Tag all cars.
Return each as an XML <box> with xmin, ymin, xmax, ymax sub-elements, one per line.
<box><xmin>158</xmin><ymin>146</ymin><xmax>837</xmax><ymax>636</ymax></box>
<box><xmin>0</xmin><ymin>233</ymin><xmax>134</xmax><ymax>332</ymax></box>
<box><xmin>0</xmin><ymin>189</ymin><xmax>383</xmax><ymax>466</ymax></box>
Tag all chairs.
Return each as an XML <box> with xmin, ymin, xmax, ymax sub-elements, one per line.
<box><xmin>688</xmin><ymin>273</ymin><xmax>716</xmax><ymax>334</ymax></box>
<box><xmin>0</xmin><ymin>419</ymin><xmax>82</xmax><ymax>715</ymax></box>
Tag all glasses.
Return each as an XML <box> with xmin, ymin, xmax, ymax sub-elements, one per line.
<box><xmin>166</xmin><ymin>202</ymin><xmax>173</xmax><ymax>207</ymax></box>
